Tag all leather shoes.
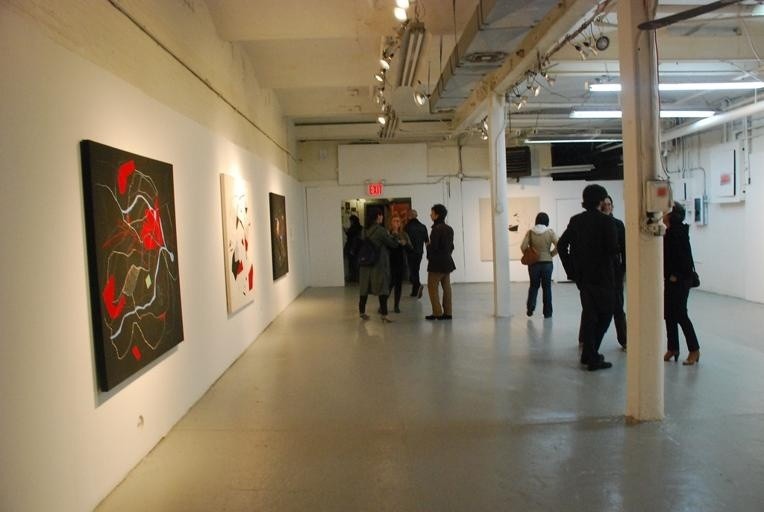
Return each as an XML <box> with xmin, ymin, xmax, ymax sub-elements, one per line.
<box><xmin>394</xmin><ymin>305</ymin><xmax>400</xmax><ymax>312</ymax></box>
<box><xmin>579</xmin><ymin>339</ymin><xmax>627</xmax><ymax>371</ymax></box>
<box><xmin>544</xmin><ymin>314</ymin><xmax>552</xmax><ymax>317</ymax></box>
<box><xmin>442</xmin><ymin>315</ymin><xmax>452</xmax><ymax>319</ymax></box>
<box><xmin>527</xmin><ymin>305</ymin><xmax>533</xmax><ymax>316</ymax></box>
<box><xmin>425</xmin><ymin>315</ymin><xmax>443</xmax><ymax>320</ymax></box>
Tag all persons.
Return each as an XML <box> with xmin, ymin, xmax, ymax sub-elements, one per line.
<box><xmin>359</xmin><ymin>208</ymin><xmax>402</xmax><ymax>323</ymax></box>
<box><xmin>343</xmin><ymin>215</ymin><xmax>363</xmax><ymax>282</ymax></box>
<box><xmin>379</xmin><ymin>213</ymin><xmax>414</xmax><ymax>314</ymax></box>
<box><xmin>425</xmin><ymin>204</ymin><xmax>456</xmax><ymax>321</ymax></box>
<box><xmin>557</xmin><ymin>184</ymin><xmax>618</xmax><ymax>371</ymax></box>
<box><xmin>664</xmin><ymin>201</ymin><xmax>700</xmax><ymax>365</ymax></box>
<box><xmin>522</xmin><ymin>213</ymin><xmax>558</xmax><ymax>318</ymax></box>
<box><xmin>405</xmin><ymin>209</ymin><xmax>428</xmax><ymax>298</ymax></box>
<box><xmin>578</xmin><ymin>196</ymin><xmax>626</xmax><ymax>352</ymax></box>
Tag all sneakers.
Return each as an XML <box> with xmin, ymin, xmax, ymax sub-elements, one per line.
<box><xmin>417</xmin><ymin>285</ymin><xmax>425</xmax><ymax>298</ymax></box>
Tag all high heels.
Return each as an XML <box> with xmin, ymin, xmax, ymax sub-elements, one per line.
<box><xmin>360</xmin><ymin>313</ymin><xmax>369</xmax><ymax>320</ymax></box>
<box><xmin>664</xmin><ymin>350</ymin><xmax>679</xmax><ymax>361</ymax></box>
<box><xmin>683</xmin><ymin>351</ymin><xmax>700</xmax><ymax>365</ymax></box>
<box><xmin>382</xmin><ymin>315</ymin><xmax>394</xmax><ymax>323</ymax></box>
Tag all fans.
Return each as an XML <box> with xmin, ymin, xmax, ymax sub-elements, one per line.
<box><xmin>637</xmin><ymin>0</ymin><xmax>764</xmax><ymax>30</ymax></box>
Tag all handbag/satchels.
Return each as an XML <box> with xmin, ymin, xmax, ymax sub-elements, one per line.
<box><xmin>521</xmin><ymin>246</ymin><xmax>539</xmax><ymax>265</ymax></box>
<box><xmin>689</xmin><ymin>271</ymin><xmax>700</xmax><ymax>287</ymax></box>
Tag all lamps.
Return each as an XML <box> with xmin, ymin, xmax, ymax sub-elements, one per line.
<box><xmin>374</xmin><ymin>0</ymin><xmax>433</xmax><ymax>140</ymax></box>
<box><xmin>477</xmin><ymin>0</ymin><xmax>615</xmax><ymax>143</ymax></box>
<box><xmin>541</xmin><ymin>164</ymin><xmax>596</xmax><ymax>174</ymax></box>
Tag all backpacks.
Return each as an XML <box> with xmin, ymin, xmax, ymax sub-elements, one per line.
<box><xmin>356</xmin><ymin>227</ymin><xmax>381</xmax><ymax>265</ymax></box>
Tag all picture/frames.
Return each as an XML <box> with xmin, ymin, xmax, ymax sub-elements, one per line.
<box><xmin>220</xmin><ymin>173</ymin><xmax>260</xmax><ymax>314</ymax></box>
<box><xmin>269</xmin><ymin>192</ymin><xmax>288</xmax><ymax>280</ymax></box>
<box><xmin>79</xmin><ymin>140</ymin><xmax>185</xmax><ymax>391</ymax></box>
